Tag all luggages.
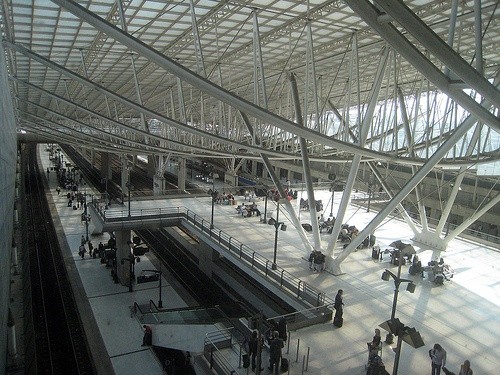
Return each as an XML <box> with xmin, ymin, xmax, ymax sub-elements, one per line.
<box><xmin>281</xmin><ymin>354</ymin><xmax>288</xmax><ymax>373</ymax></box>
<box><xmin>333</xmin><ymin>303</ymin><xmax>344</xmax><ymax>328</ymax></box>
<box><xmin>243</xmin><ymin>353</ymin><xmax>250</xmax><ymax>368</ymax></box>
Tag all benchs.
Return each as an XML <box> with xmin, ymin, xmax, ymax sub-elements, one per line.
<box><xmin>235</xmin><ymin>205</ymin><xmax>257</xmax><ymax>216</ymax></box>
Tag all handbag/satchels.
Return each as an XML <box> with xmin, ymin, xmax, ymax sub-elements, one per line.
<box><xmin>429</xmin><ymin>349</ymin><xmax>435</xmax><ymax>360</ymax></box>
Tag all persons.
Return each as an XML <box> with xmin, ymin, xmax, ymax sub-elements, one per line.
<box><xmin>80</xmin><ymin>201</ymin><xmax>83</xmax><ymax>210</ymax></box>
<box><xmin>367</xmin><ymin>329</ymin><xmax>381</xmax><ymax>364</ymax></box>
<box><xmin>459</xmin><ymin>360</ymin><xmax>473</xmax><ymax>375</ymax></box>
<box><xmin>477</xmin><ymin>224</ymin><xmax>483</xmax><ymax>236</ymax></box>
<box><xmin>362</xmin><ymin>236</ymin><xmax>374</xmax><ymax>247</ymax></box>
<box><xmin>78</xmin><ymin>234</ymin><xmax>103</xmax><ymax>259</ymax></box>
<box><xmin>318</xmin><ymin>214</ymin><xmax>336</xmax><ymax>230</ymax></box>
<box><xmin>430</xmin><ymin>344</ymin><xmax>446</xmax><ymax>375</ymax></box>
<box><xmin>108</xmin><ymin>237</ymin><xmax>115</xmax><ymax>249</ymax></box>
<box><xmin>112</xmin><ymin>272</ymin><xmax>118</xmax><ymax>280</ymax></box>
<box><xmin>451</xmin><ymin>217</ymin><xmax>462</xmax><ymax>229</ymax></box>
<box><xmin>270</xmin><ymin>187</ymin><xmax>297</xmax><ymax>202</ymax></box>
<box><xmin>309</xmin><ymin>251</ymin><xmax>326</xmax><ymax>264</ymax></box>
<box><xmin>338</xmin><ymin>225</ymin><xmax>358</xmax><ymax>243</ymax></box>
<box><xmin>333</xmin><ymin>289</ymin><xmax>344</xmax><ymax>326</ymax></box>
<box><xmin>141</xmin><ymin>325</ymin><xmax>152</xmax><ymax>345</ymax></box>
<box><xmin>56</xmin><ymin>174</ymin><xmax>75</xmax><ymax>206</ymax></box>
<box><xmin>249</xmin><ymin>329</ymin><xmax>284</xmax><ymax>371</ymax></box>
<box><xmin>235</xmin><ymin>201</ymin><xmax>257</xmax><ymax>217</ymax></box>
<box><xmin>208</xmin><ymin>189</ymin><xmax>234</xmax><ymax>204</ymax></box>
<box><xmin>81</xmin><ymin>213</ymin><xmax>91</xmax><ymax>221</ymax></box>
<box><xmin>413</xmin><ymin>255</ymin><xmax>444</xmax><ymax>278</ymax></box>
<box><xmin>471</xmin><ymin>223</ymin><xmax>476</xmax><ymax>234</ymax></box>
